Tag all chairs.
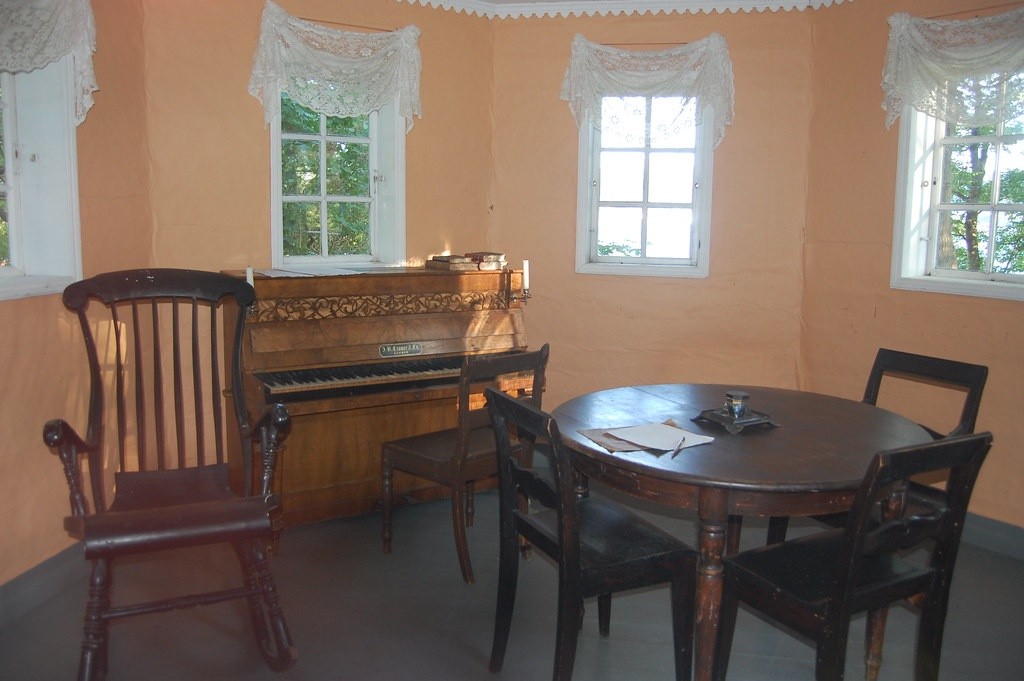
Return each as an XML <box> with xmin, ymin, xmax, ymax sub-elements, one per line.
<box><xmin>486</xmin><ymin>388</ymin><xmax>696</xmax><ymax>681</ymax></box>
<box><xmin>768</xmin><ymin>346</ymin><xmax>989</xmax><ymax>546</ymax></box>
<box><xmin>43</xmin><ymin>267</ymin><xmax>299</xmax><ymax>681</ymax></box>
<box><xmin>383</xmin><ymin>343</ymin><xmax>550</xmax><ymax>583</ymax></box>
<box><xmin>712</xmin><ymin>430</ymin><xmax>994</xmax><ymax>681</ymax></box>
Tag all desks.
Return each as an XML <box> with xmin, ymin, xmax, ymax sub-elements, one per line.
<box><xmin>548</xmin><ymin>384</ymin><xmax>934</xmax><ymax>681</ymax></box>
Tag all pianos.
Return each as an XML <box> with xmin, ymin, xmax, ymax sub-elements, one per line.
<box><xmin>217</xmin><ymin>266</ymin><xmax>548</xmax><ymax>557</ymax></box>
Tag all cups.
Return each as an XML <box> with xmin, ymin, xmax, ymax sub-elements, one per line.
<box><xmin>721</xmin><ymin>391</ymin><xmax>751</xmax><ymax>419</ymax></box>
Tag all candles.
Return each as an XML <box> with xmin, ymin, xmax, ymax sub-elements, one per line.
<box><xmin>247</xmin><ymin>265</ymin><xmax>254</xmax><ymax>285</ymax></box>
<box><xmin>522</xmin><ymin>259</ymin><xmax>529</xmax><ymax>290</ymax></box>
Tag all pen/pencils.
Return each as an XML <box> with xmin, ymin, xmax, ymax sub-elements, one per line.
<box><xmin>671</xmin><ymin>436</ymin><xmax>685</xmax><ymax>459</ymax></box>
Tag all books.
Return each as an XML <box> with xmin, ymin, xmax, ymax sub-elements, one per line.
<box><xmin>426</xmin><ymin>252</ymin><xmax>507</xmax><ymax>270</ymax></box>
